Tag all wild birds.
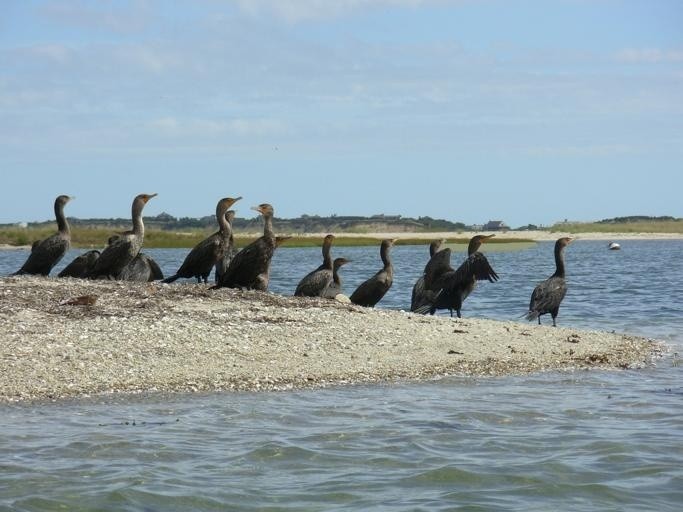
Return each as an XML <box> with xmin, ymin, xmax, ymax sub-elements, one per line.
<box><xmin>294</xmin><ymin>234</ymin><xmax>335</xmax><ymax>297</ymax></box>
<box><xmin>349</xmin><ymin>238</ymin><xmax>399</xmax><ymax>308</ymax></box>
<box><xmin>410</xmin><ymin>237</ymin><xmax>447</xmax><ymax>315</ymax></box>
<box><xmin>160</xmin><ymin>196</ymin><xmax>243</xmax><ymax>283</ymax></box>
<box><xmin>58</xmin><ymin>192</ymin><xmax>164</xmax><ymax>281</ymax></box>
<box><xmin>324</xmin><ymin>258</ymin><xmax>355</xmax><ymax>299</ymax></box>
<box><xmin>414</xmin><ymin>233</ymin><xmax>500</xmax><ymax>319</ymax></box>
<box><xmin>252</xmin><ymin>236</ymin><xmax>293</xmax><ymax>290</ymax></box>
<box><xmin>207</xmin><ymin>203</ymin><xmax>276</xmax><ymax>291</ymax></box>
<box><xmin>9</xmin><ymin>194</ymin><xmax>76</xmax><ymax>277</ymax></box>
<box><xmin>518</xmin><ymin>237</ymin><xmax>576</xmax><ymax>327</ymax></box>
<box><xmin>215</xmin><ymin>209</ymin><xmax>239</xmax><ymax>284</ymax></box>
